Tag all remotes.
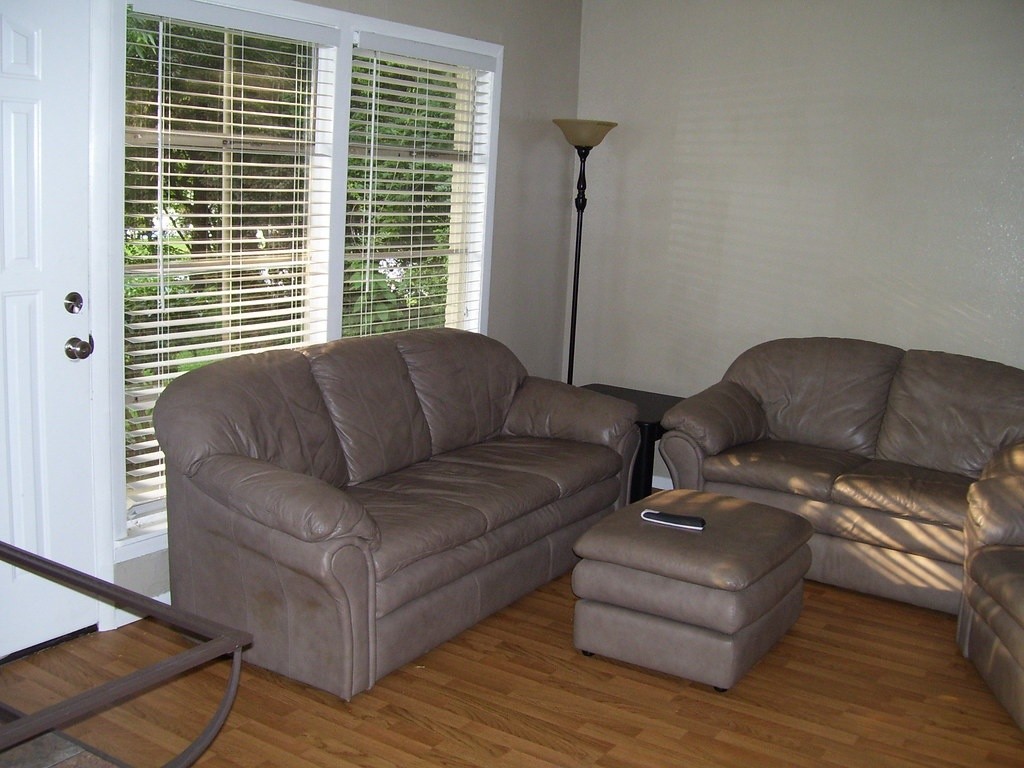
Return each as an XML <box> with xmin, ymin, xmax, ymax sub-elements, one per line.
<box><xmin>640</xmin><ymin>509</ymin><xmax>707</xmax><ymax>530</ymax></box>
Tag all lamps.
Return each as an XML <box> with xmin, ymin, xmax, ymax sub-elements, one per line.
<box><xmin>552</xmin><ymin>117</ymin><xmax>618</xmax><ymax>385</ymax></box>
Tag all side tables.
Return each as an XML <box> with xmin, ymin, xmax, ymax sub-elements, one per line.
<box><xmin>577</xmin><ymin>384</ymin><xmax>687</xmax><ymax>504</ymax></box>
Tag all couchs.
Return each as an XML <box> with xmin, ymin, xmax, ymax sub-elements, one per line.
<box><xmin>153</xmin><ymin>327</ymin><xmax>642</xmax><ymax>702</ymax></box>
<box><xmin>954</xmin><ymin>476</ymin><xmax>1024</xmax><ymax>733</ymax></box>
<box><xmin>658</xmin><ymin>337</ymin><xmax>1024</xmax><ymax>617</ymax></box>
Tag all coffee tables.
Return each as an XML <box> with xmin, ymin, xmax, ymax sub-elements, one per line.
<box><xmin>0</xmin><ymin>541</ymin><xmax>254</xmax><ymax>768</ymax></box>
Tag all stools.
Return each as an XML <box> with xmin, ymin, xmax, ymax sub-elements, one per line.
<box><xmin>570</xmin><ymin>489</ymin><xmax>815</xmax><ymax>693</ymax></box>
